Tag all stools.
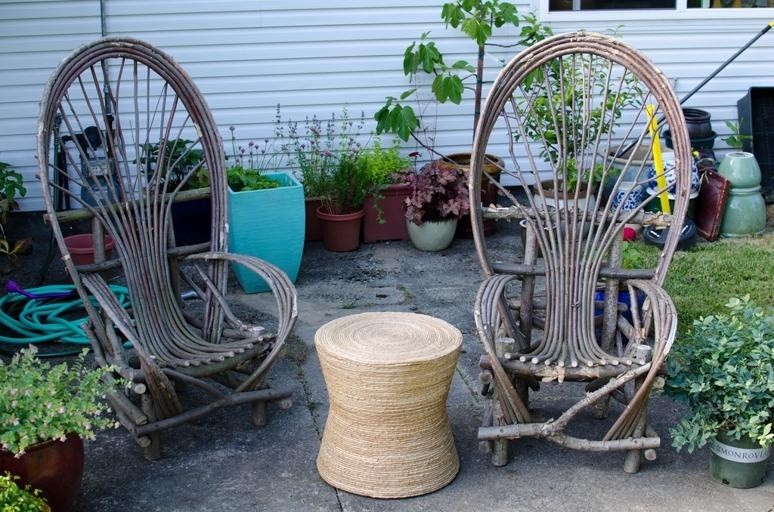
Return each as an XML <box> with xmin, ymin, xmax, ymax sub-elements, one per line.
<box><xmin>313</xmin><ymin>311</ymin><xmax>464</xmax><ymax>498</ymax></box>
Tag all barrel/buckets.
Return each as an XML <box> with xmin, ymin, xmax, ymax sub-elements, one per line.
<box><xmin>65</xmin><ymin>232</ymin><xmax>115</xmax><ymax>264</ymax></box>
<box><xmin>675</xmin><ymin>107</ymin><xmax>711</xmax><ymax>136</ymax></box>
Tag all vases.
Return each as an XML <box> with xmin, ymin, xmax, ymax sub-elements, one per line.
<box><xmin>0</xmin><ymin>431</ymin><xmax>84</xmax><ymax>512</ymax></box>
<box><xmin>605</xmin><ymin>109</ymin><xmax>766</xmax><ymax>237</ymax></box>
<box><xmin>64</xmin><ymin>234</ymin><xmax>113</xmax><ymax>280</ymax></box>
<box><xmin>406</xmin><ymin>219</ymin><xmax>458</xmax><ymax>251</ymax></box>
<box><xmin>224</xmin><ymin>171</ymin><xmax>306</xmax><ymax>294</ymax></box>
<box><xmin>520</xmin><ymin>219</ymin><xmax>555</xmax><ymax>256</ymax></box>
<box><xmin>317</xmin><ymin>201</ymin><xmax>366</xmax><ymax>251</ymax></box>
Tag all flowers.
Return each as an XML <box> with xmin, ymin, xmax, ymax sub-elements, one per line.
<box><xmin>0</xmin><ymin>345</ymin><xmax>132</xmax><ymax>458</ymax></box>
<box><xmin>405</xmin><ymin>160</ymin><xmax>470</xmax><ymax>224</ymax></box>
<box><xmin>219</xmin><ymin>123</ymin><xmax>304</xmax><ymax>192</ymax></box>
<box><xmin>274</xmin><ymin>102</ymin><xmax>372</xmax><ymax>210</ymax></box>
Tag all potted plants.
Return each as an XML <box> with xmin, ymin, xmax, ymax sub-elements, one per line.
<box><xmin>361</xmin><ymin>140</ymin><xmax>408</xmax><ymax>240</ymax></box>
<box><xmin>666</xmin><ymin>293</ymin><xmax>774</xmax><ymax>487</ymax></box>
<box><xmin>512</xmin><ymin>57</ymin><xmax>645</xmax><ymax>241</ymax></box>
<box><xmin>0</xmin><ymin>471</ymin><xmax>51</xmax><ymax>512</ymax></box>
<box><xmin>0</xmin><ymin>163</ymin><xmax>32</xmax><ymax>265</ymax></box>
<box><xmin>134</xmin><ymin>138</ymin><xmax>210</xmax><ymax>259</ymax></box>
<box><xmin>372</xmin><ymin>0</ymin><xmax>551</xmax><ymax>239</ymax></box>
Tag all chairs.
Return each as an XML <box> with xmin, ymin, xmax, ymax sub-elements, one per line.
<box><xmin>35</xmin><ymin>35</ymin><xmax>297</xmax><ymax>459</ymax></box>
<box><xmin>468</xmin><ymin>33</ymin><xmax>691</xmax><ymax>473</ymax></box>
